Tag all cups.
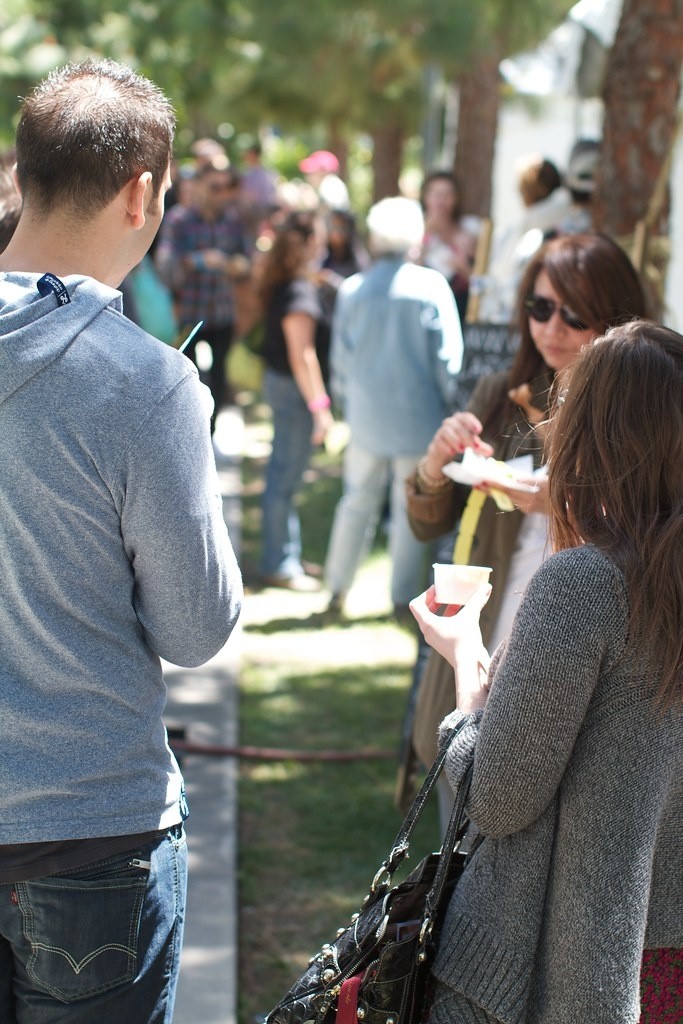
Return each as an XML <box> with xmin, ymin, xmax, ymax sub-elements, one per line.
<box><xmin>432</xmin><ymin>563</ymin><xmax>493</xmax><ymax>607</ymax></box>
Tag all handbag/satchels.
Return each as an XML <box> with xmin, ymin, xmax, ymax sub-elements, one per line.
<box><xmin>262</xmin><ymin>713</ymin><xmax>485</xmax><ymax>1024</ymax></box>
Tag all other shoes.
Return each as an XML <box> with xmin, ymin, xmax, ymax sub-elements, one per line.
<box><xmin>262</xmin><ymin>573</ymin><xmax>320</xmax><ymax>591</ymax></box>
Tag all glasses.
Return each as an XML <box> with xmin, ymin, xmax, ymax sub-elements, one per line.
<box><xmin>206</xmin><ymin>180</ymin><xmax>237</xmax><ymax>191</ymax></box>
<box><xmin>524</xmin><ymin>292</ymin><xmax>592</xmax><ymax>330</ymax></box>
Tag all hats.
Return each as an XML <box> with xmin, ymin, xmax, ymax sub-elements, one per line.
<box><xmin>299</xmin><ymin>151</ymin><xmax>339</xmax><ymax>173</ymax></box>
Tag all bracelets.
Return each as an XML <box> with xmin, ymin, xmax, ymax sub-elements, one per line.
<box><xmin>418</xmin><ymin>456</ymin><xmax>450</xmax><ymax>488</ymax></box>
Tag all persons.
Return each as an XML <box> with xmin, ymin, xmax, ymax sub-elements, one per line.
<box><xmin>408</xmin><ymin>318</ymin><xmax>682</xmax><ymax>1024</ymax></box>
<box><xmin>407</xmin><ymin>229</ymin><xmax>651</xmax><ymax>845</ymax></box>
<box><xmin>2</xmin><ymin>132</ymin><xmax>592</xmax><ymax>631</ymax></box>
<box><xmin>0</xmin><ymin>60</ymin><xmax>245</xmax><ymax>1024</ymax></box>
